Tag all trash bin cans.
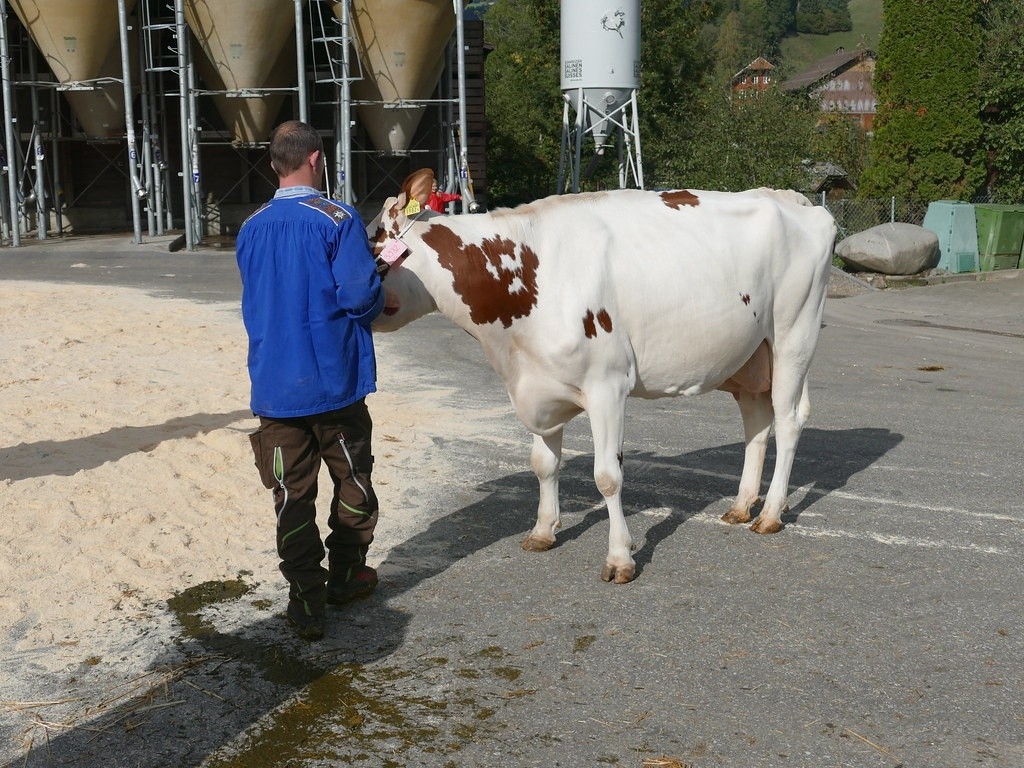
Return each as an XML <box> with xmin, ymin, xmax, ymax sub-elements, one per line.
<box><xmin>974</xmin><ymin>203</ymin><xmax>1024</xmax><ymax>271</ymax></box>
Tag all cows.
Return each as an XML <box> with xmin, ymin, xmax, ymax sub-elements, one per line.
<box><xmin>365</xmin><ymin>186</ymin><xmax>840</xmax><ymax>586</ymax></box>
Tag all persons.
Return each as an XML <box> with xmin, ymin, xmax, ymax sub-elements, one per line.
<box><xmin>420</xmin><ymin>179</ymin><xmax>463</xmax><ymax>214</ymax></box>
<box><xmin>236</xmin><ymin>119</ymin><xmax>388</xmax><ymax>642</ymax></box>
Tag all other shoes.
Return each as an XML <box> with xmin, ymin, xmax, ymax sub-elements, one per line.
<box><xmin>288</xmin><ymin>601</ymin><xmax>323</xmax><ymax>641</ymax></box>
<box><xmin>328</xmin><ymin>565</ymin><xmax>377</xmax><ymax>603</ymax></box>
